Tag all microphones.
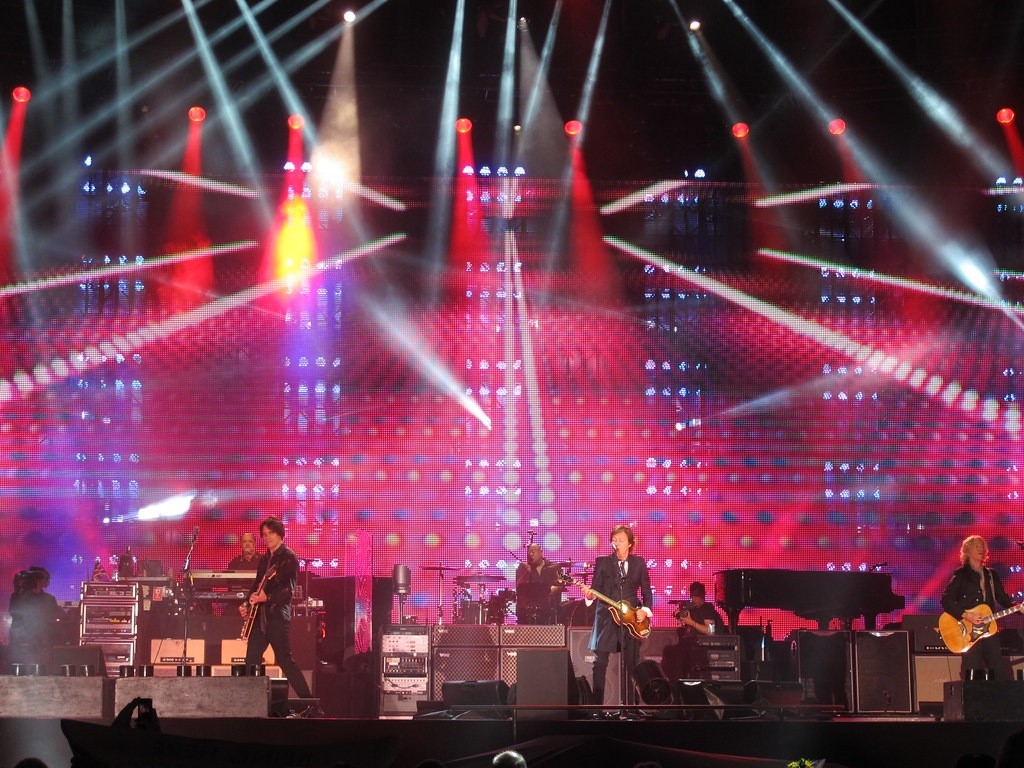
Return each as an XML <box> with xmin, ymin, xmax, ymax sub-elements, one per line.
<box><xmin>611</xmin><ymin>542</ymin><xmax>617</xmax><ymax>552</ymax></box>
<box><xmin>192</xmin><ymin>526</ymin><xmax>201</xmax><ymax>540</ymax></box>
<box><xmin>876</xmin><ymin>562</ymin><xmax>888</xmax><ymax>566</ymax></box>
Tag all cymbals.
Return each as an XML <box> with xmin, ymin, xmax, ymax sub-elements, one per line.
<box><xmin>453</xmin><ymin>575</ymin><xmax>506</xmax><ymax>583</ymax></box>
<box><xmin>419</xmin><ymin>566</ymin><xmax>462</xmax><ymax>571</ymax></box>
<box><xmin>548</xmin><ymin>561</ymin><xmax>579</xmax><ymax>569</ymax></box>
<box><xmin>568</xmin><ymin>572</ymin><xmax>594</xmax><ymax>577</ymax></box>
<box><xmin>572</xmin><ymin>561</ymin><xmax>596</xmax><ymax>567</ymax></box>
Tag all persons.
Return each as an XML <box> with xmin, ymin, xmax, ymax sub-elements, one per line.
<box><xmin>222</xmin><ymin>533</ymin><xmax>266</xmax><ymax>617</ymax></box>
<box><xmin>941</xmin><ymin>535</ymin><xmax>1024</xmax><ymax>680</ymax></box>
<box><xmin>673</xmin><ymin>582</ymin><xmax>724</xmax><ymax>641</ymax></box>
<box><xmin>516</xmin><ymin>544</ymin><xmax>561</xmax><ymax>626</ymax></box>
<box><xmin>490</xmin><ymin>750</ymin><xmax>527</xmax><ymax>768</ymax></box>
<box><xmin>9</xmin><ymin>566</ymin><xmax>82</xmax><ymax>675</ymax></box>
<box><xmin>957</xmin><ymin>728</ymin><xmax>1024</xmax><ymax>768</ymax></box>
<box><xmin>112</xmin><ymin>697</ymin><xmax>160</xmax><ymax>732</ymax></box>
<box><xmin>238</xmin><ymin>517</ymin><xmax>324</xmax><ymax>717</ymax></box>
<box><xmin>584</xmin><ymin>525</ymin><xmax>653</xmax><ymax>718</ymax></box>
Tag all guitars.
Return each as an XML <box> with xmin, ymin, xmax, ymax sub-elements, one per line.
<box><xmin>937</xmin><ymin>602</ymin><xmax>1024</xmax><ymax>655</ymax></box>
<box><xmin>239</xmin><ymin>559</ymin><xmax>279</xmax><ymax>644</ymax></box>
<box><xmin>556</xmin><ymin>574</ymin><xmax>651</xmax><ymax>640</ymax></box>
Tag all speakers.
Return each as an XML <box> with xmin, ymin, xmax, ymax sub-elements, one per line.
<box><xmin>221</xmin><ymin>639</ymin><xmax>276</xmax><ymax>665</ymax></box>
<box><xmin>856</xmin><ymin>630</ymin><xmax>912</xmax><ymax>713</ymax></box>
<box><xmin>150</xmin><ymin>639</ymin><xmax>206</xmax><ymax>664</ymax></box>
<box><xmin>634</xmin><ymin>626</ymin><xmax>684</xmax><ymax>711</ymax></box>
<box><xmin>911</xmin><ymin>628</ymin><xmax>952</xmax><ymax>654</ymax></box>
<box><xmin>431</xmin><ymin>624</ymin><xmax>621</xmax><ymax>720</ymax></box>
<box><xmin>913</xmin><ymin>653</ymin><xmax>964</xmax><ymax>713</ymax></box>
<box><xmin>795</xmin><ymin>628</ymin><xmax>854</xmax><ymax>713</ymax></box>
<box><xmin>0</xmin><ymin>674</ymin><xmax>116</xmax><ymax>720</ymax></box>
<box><xmin>314</xmin><ymin>671</ymin><xmax>380</xmax><ymax>718</ymax></box>
<box><xmin>943</xmin><ymin>679</ymin><xmax>1024</xmax><ymax>721</ymax></box>
<box><xmin>115</xmin><ymin>676</ymin><xmax>271</xmax><ymax>717</ymax></box>
<box><xmin>311</xmin><ymin>575</ymin><xmax>356</xmax><ymax>666</ymax></box>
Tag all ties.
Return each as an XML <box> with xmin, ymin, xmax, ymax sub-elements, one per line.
<box><xmin>620</xmin><ymin>559</ymin><xmax>626</xmax><ymax>577</ymax></box>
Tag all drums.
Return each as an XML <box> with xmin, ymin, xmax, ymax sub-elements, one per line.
<box><xmin>547</xmin><ymin>598</ymin><xmax>596</xmax><ymax>627</ymax></box>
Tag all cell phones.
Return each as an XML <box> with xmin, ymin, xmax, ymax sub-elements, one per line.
<box><xmin>138</xmin><ymin>698</ymin><xmax>153</xmax><ymax>717</ymax></box>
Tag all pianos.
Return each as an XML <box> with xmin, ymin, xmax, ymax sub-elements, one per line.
<box><xmin>710</xmin><ymin>568</ymin><xmax>906</xmax><ymax>636</ymax></box>
